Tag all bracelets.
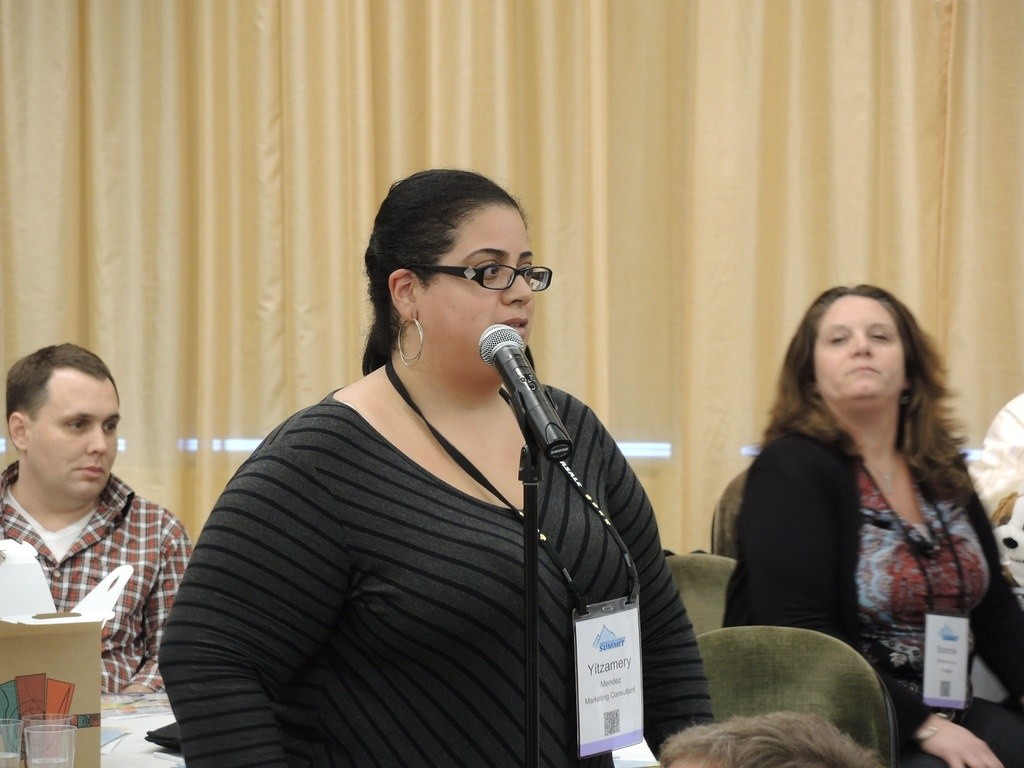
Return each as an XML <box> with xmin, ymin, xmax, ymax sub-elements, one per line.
<box><xmin>915</xmin><ymin>712</ymin><xmax>947</xmax><ymax>741</ymax></box>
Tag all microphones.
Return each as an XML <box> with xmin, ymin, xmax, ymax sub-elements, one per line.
<box><xmin>477</xmin><ymin>324</ymin><xmax>573</xmax><ymax>464</ymax></box>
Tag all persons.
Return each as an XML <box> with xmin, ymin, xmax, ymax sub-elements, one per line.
<box><xmin>658</xmin><ymin>710</ymin><xmax>885</xmax><ymax>768</ymax></box>
<box><xmin>0</xmin><ymin>342</ymin><xmax>194</xmax><ymax>695</ymax></box>
<box><xmin>158</xmin><ymin>168</ymin><xmax>715</xmax><ymax>768</ymax></box>
<box><xmin>968</xmin><ymin>392</ymin><xmax>1024</xmax><ymax>523</ymax></box>
<box><xmin>720</xmin><ymin>285</ymin><xmax>1024</xmax><ymax>768</ymax></box>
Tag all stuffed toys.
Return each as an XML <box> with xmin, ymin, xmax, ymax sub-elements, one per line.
<box><xmin>988</xmin><ymin>490</ymin><xmax>1024</xmax><ymax>587</ymax></box>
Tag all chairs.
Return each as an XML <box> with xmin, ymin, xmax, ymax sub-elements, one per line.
<box><xmin>664</xmin><ymin>471</ymin><xmax>898</xmax><ymax>768</ymax></box>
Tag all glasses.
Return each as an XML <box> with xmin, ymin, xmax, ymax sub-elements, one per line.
<box><xmin>401</xmin><ymin>263</ymin><xmax>553</xmax><ymax>292</ymax></box>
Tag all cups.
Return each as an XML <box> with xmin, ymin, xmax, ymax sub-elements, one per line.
<box><xmin>22</xmin><ymin>714</ymin><xmax>75</xmax><ymax>727</ymax></box>
<box><xmin>0</xmin><ymin>718</ymin><xmax>24</xmax><ymax>768</ymax></box>
<box><xmin>24</xmin><ymin>724</ymin><xmax>77</xmax><ymax>768</ymax></box>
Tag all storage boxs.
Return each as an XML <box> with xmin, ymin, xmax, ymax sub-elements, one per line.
<box><xmin>0</xmin><ymin>538</ymin><xmax>134</xmax><ymax>768</ymax></box>
